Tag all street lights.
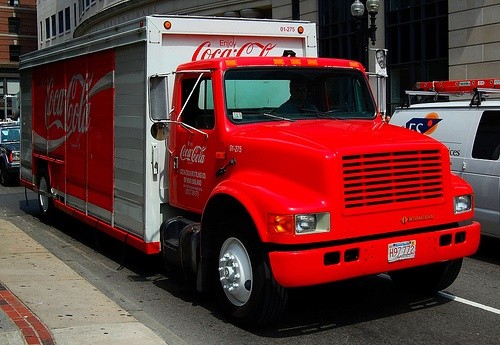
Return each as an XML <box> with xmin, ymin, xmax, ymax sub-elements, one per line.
<box><xmin>350</xmin><ymin>0</ymin><xmax>380</xmax><ymax>45</ymax></box>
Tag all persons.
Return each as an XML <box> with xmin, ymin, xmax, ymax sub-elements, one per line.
<box><xmin>377</xmin><ymin>50</ymin><xmax>387</xmax><ymax>77</ymax></box>
<box><xmin>270</xmin><ymin>77</ymin><xmax>318</xmax><ymax>115</ymax></box>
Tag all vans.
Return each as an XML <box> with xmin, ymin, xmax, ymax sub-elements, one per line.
<box><xmin>386</xmin><ymin>79</ymin><xmax>500</xmax><ymax>238</ymax></box>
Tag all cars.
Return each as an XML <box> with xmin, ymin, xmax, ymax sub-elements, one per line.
<box><xmin>0</xmin><ymin>121</ymin><xmax>21</xmax><ymax>186</ymax></box>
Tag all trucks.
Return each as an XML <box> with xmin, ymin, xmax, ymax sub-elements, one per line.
<box><xmin>17</xmin><ymin>14</ymin><xmax>482</xmax><ymax>326</ymax></box>
